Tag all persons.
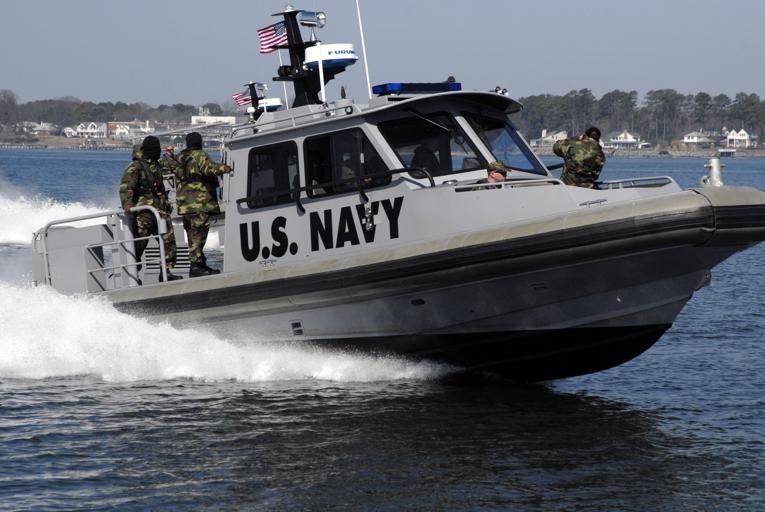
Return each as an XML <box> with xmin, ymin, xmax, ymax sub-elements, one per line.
<box><xmin>158</xmin><ymin>145</ymin><xmax>177</xmax><ymax>175</ymax></box>
<box><xmin>470</xmin><ymin>161</ymin><xmax>512</xmax><ymax>190</ymax></box>
<box><xmin>410</xmin><ymin>132</ymin><xmax>443</xmax><ymax>179</ymax></box>
<box><xmin>173</xmin><ymin>132</ymin><xmax>234</xmax><ymax>277</ymax></box>
<box><xmin>118</xmin><ymin>136</ymin><xmax>182</xmax><ymax>285</ymax></box>
<box><xmin>553</xmin><ymin>127</ymin><xmax>604</xmax><ymax>190</ymax></box>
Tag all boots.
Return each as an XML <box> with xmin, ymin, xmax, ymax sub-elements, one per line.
<box><xmin>159</xmin><ymin>268</ymin><xmax>183</xmax><ymax>282</ymax></box>
<box><xmin>189</xmin><ymin>262</ymin><xmax>220</xmax><ymax>277</ymax></box>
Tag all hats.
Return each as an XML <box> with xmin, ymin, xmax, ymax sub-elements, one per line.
<box><xmin>487</xmin><ymin>161</ymin><xmax>512</xmax><ymax>173</ymax></box>
<box><xmin>186</xmin><ymin>132</ymin><xmax>202</xmax><ymax>148</ymax></box>
<box><xmin>143</xmin><ymin>137</ymin><xmax>161</xmax><ymax>160</ymax></box>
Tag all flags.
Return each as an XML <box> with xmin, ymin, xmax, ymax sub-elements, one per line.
<box><xmin>233</xmin><ymin>91</ymin><xmax>252</xmax><ymax>106</ymax></box>
<box><xmin>257</xmin><ymin>21</ymin><xmax>289</xmax><ymax>55</ymax></box>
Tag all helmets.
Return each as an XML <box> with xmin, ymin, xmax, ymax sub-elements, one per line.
<box><xmin>586</xmin><ymin>128</ymin><xmax>600</xmax><ymax>142</ymax></box>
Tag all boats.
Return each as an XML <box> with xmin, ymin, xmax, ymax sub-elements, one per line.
<box><xmin>30</xmin><ymin>0</ymin><xmax>765</xmax><ymax>382</ymax></box>
